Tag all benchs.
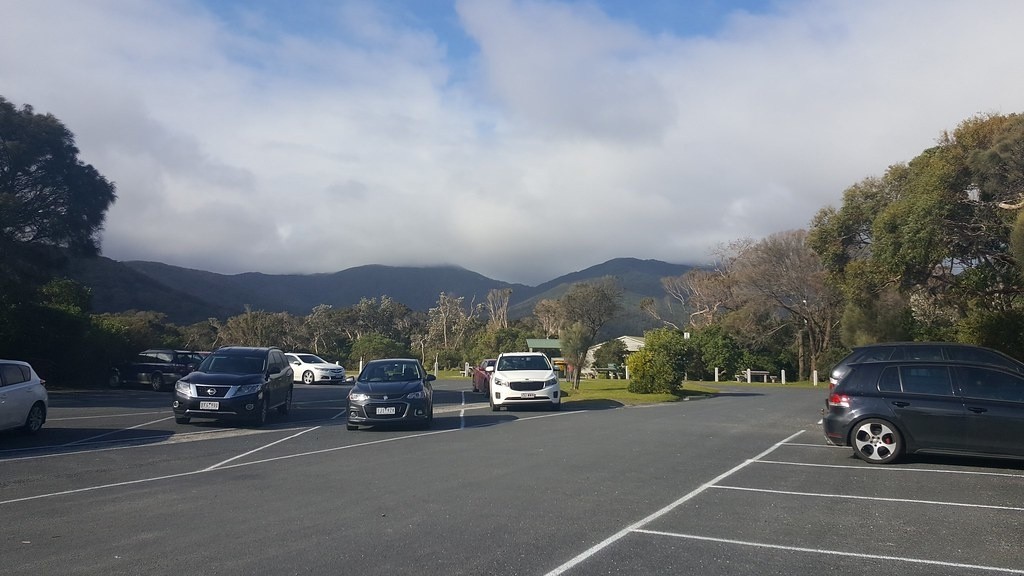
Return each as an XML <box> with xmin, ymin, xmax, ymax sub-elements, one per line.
<box><xmin>735</xmin><ymin>374</ymin><xmax>745</xmax><ymax>382</ymax></box>
<box><xmin>617</xmin><ymin>373</ymin><xmax>622</xmax><ymax>379</ymax></box>
<box><xmin>770</xmin><ymin>376</ymin><xmax>778</xmax><ymax>383</ymax></box>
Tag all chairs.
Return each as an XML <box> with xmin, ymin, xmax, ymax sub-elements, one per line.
<box><xmin>404</xmin><ymin>367</ymin><xmax>414</xmax><ymax>377</ymax></box>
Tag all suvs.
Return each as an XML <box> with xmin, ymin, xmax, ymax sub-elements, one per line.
<box><xmin>485</xmin><ymin>351</ymin><xmax>561</xmax><ymax>412</ymax></box>
<box><xmin>829</xmin><ymin>344</ymin><xmax>1024</xmax><ymax>396</ymax></box>
<box><xmin>173</xmin><ymin>345</ymin><xmax>294</xmax><ymax>426</ymax></box>
<box><xmin>174</xmin><ymin>350</ymin><xmax>203</xmax><ymax>375</ymax></box>
<box><xmin>108</xmin><ymin>349</ymin><xmax>190</xmax><ymax>391</ymax></box>
<box><xmin>822</xmin><ymin>360</ymin><xmax>1024</xmax><ymax>465</ymax></box>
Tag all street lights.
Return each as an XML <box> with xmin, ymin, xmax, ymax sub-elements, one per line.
<box><xmin>684</xmin><ymin>332</ymin><xmax>691</xmax><ymax>383</ymax></box>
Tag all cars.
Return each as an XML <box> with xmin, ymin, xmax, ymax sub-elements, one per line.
<box><xmin>0</xmin><ymin>360</ymin><xmax>48</xmax><ymax>434</ymax></box>
<box><xmin>345</xmin><ymin>358</ymin><xmax>436</xmax><ymax>430</ymax></box>
<box><xmin>469</xmin><ymin>359</ymin><xmax>497</xmax><ymax>398</ymax></box>
<box><xmin>284</xmin><ymin>353</ymin><xmax>345</xmax><ymax>384</ymax></box>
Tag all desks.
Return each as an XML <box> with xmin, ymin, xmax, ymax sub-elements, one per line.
<box><xmin>741</xmin><ymin>371</ymin><xmax>770</xmax><ymax>383</ymax></box>
<box><xmin>591</xmin><ymin>368</ymin><xmax>616</xmax><ymax>380</ymax></box>
